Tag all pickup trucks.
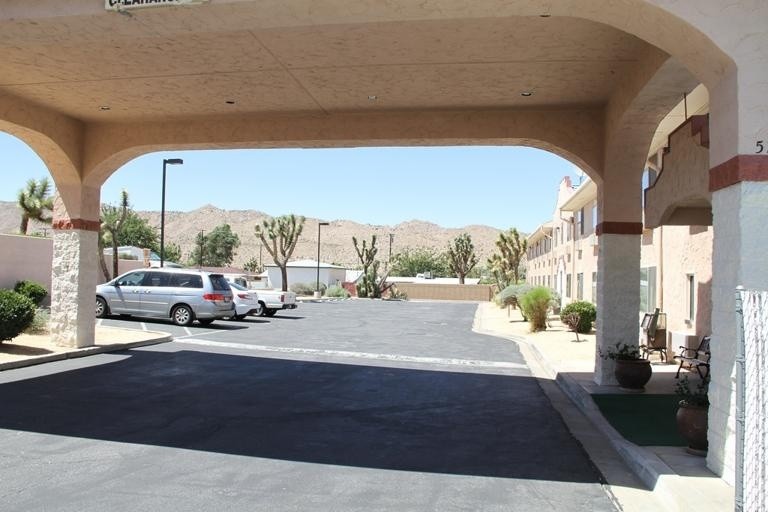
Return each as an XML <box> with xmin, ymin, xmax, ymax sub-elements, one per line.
<box><xmin>223</xmin><ymin>272</ymin><xmax>298</xmax><ymax>318</ymax></box>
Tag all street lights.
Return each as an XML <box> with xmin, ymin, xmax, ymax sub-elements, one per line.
<box><xmin>199</xmin><ymin>229</ymin><xmax>205</xmax><ymax>267</ymax></box>
<box><xmin>160</xmin><ymin>158</ymin><xmax>184</xmax><ymax>267</ymax></box>
<box><xmin>313</xmin><ymin>222</ymin><xmax>329</xmax><ymax>299</ymax></box>
<box><xmin>389</xmin><ymin>233</ymin><xmax>396</xmax><ymax>258</ymax></box>
<box><xmin>253</xmin><ymin>232</ymin><xmax>263</xmax><ymax>274</ymax></box>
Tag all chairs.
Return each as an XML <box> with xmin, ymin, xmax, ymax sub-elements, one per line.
<box><xmin>672</xmin><ymin>335</ymin><xmax>711</xmax><ymax>385</ymax></box>
<box><xmin>158</xmin><ymin>276</ymin><xmax>202</xmax><ymax>288</ymax></box>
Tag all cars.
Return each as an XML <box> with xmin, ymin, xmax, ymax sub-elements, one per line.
<box><xmin>95</xmin><ymin>267</ymin><xmax>259</xmax><ymax>327</ymax></box>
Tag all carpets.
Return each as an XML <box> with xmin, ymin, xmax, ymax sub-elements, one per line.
<box><xmin>590</xmin><ymin>392</ymin><xmax>694</xmax><ymax>447</ymax></box>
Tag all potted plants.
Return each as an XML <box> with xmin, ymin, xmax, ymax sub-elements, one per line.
<box><xmin>671</xmin><ymin>375</ymin><xmax>711</xmax><ymax>457</ymax></box>
<box><xmin>598</xmin><ymin>341</ymin><xmax>668</xmax><ymax>392</ymax></box>
